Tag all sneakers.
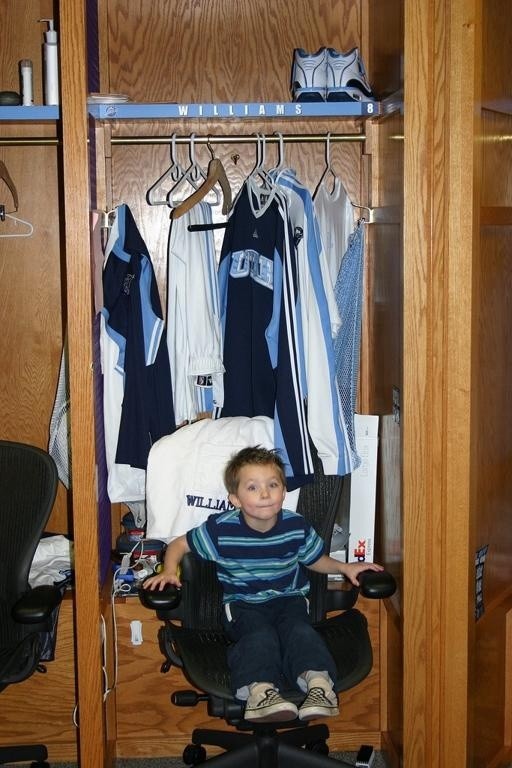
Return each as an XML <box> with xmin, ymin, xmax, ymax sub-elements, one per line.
<box><xmin>327</xmin><ymin>46</ymin><xmax>375</xmax><ymax>102</ymax></box>
<box><xmin>289</xmin><ymin>44</ymin><xmax>328</xmax><ymax>102</ymax></box>
<box><xmin>241</xmin><ymin>681</ymin><xmax>299</xmax><ymax>725</ymax></box>
<box><xmin>299</xmin><ymin>684</ymin><xmax>342</xmax><ymax>721</ymax></box>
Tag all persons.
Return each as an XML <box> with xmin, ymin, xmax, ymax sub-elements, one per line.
<box><xmin>143</xmin><ymin>443</ymin><xmax>386</xmax><ymax>722</ymax></box>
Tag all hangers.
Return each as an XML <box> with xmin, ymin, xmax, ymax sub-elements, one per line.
<box><xmin>0</xmin><ymin>158</ymin><xmax>32</xmax><ymax>218</ymax></box>
<box><xmin>136</xmin><ymin>125</ymin><xmax>377</xmax><ymax>231</ymax></box>
<box><xmin>0</xmin><ymin>204</ymin><xmax>35</xmax><ymax>239</ymax></box>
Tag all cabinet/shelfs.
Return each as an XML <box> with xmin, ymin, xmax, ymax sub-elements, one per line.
<box><xmin>1</xmin><ymin>0</ymin><xmax>406</xmax><ymax>768</ymax></box>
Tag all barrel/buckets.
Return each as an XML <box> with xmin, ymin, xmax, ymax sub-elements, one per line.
<box><xmin>115</xmin><ymin>530</ymin><xmax>158</xmax><ymax>564</ymax></box>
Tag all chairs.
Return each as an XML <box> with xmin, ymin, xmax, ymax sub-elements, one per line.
<box><xmin>136</xmin><ymin>409</ymin><xmax>398</xmax><ymax>766</ymax></box>
<box><xmin>0</xmin><ymin>437</ymin><xmax>73</xmax><ymax>768</ymax></box>
